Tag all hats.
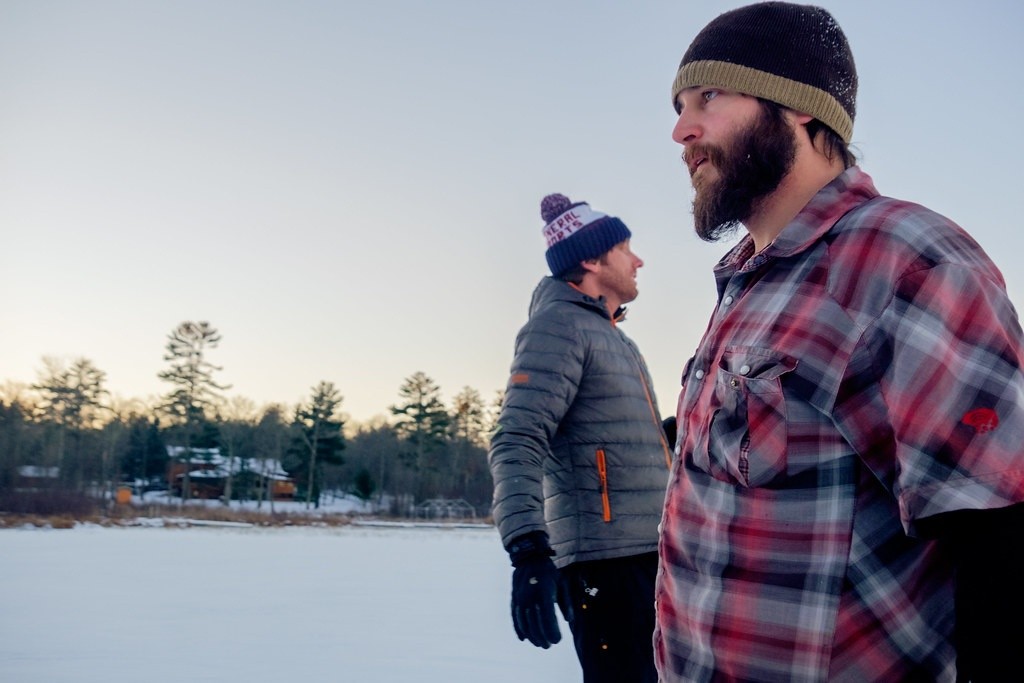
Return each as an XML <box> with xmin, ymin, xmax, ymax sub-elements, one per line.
<box><xmin>540</xmin><ymin>193</ymin><xmax>632</xmax><ymax>278</ymax></box>
<box><xmin>670</xmin><ymin>1</ymin><xmax>858</xmax><ymax>145</ymax></box>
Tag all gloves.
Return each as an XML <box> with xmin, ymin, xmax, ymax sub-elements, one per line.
<box><xmin>662</xmin><ymin>416</ymin><xmax>677</xmax><ymax>451</ymax></box>
<box><xmin>507</xmin><ymin>530</ymin><xmax>574</xmax><ymax>648</ymax></box>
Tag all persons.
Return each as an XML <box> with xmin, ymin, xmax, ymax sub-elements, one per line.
<box><xmin>651</xmin><ymin>0</ymin><xmax>1024</xmax><ymax>683</ymax></box>
<box><xmin>489</xmin><ymin>193</ymin><xmax>672</xmax><ymax>683</ymax></box>
